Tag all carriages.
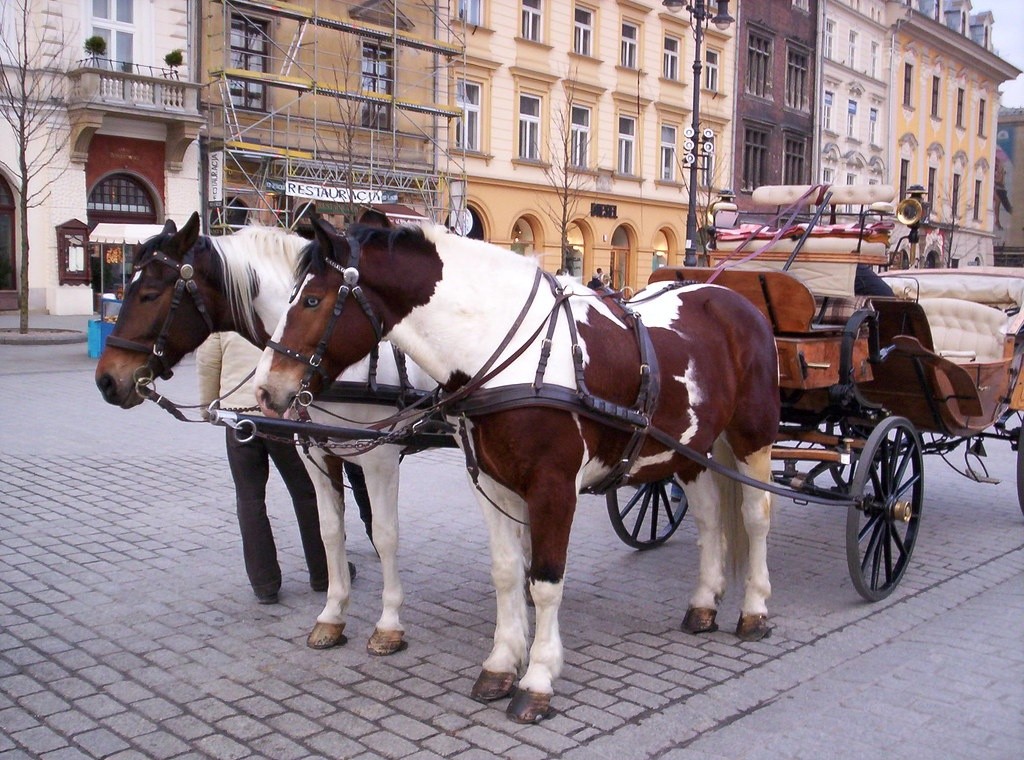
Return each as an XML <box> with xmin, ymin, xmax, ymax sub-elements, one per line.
<box><xmin>93</xmin><ymin>172</ymin><xmax>1024</xmax><ymax>727</ymax></box>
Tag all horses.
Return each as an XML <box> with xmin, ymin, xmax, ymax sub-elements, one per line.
<box><xmin>252</xmin><ymin>204</ymin><xmax>784</xmax><ymax>725</ymax></box>
<box><xmin>93</xmin><ymin>211</ymin><xmax>443</xmax><ymax>658</ymax></box>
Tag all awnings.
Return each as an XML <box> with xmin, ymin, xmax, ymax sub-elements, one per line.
<box><xmin>360</xmin><ymin>203</ymin><xmax>429</xmax><ymax>227</ymax></box>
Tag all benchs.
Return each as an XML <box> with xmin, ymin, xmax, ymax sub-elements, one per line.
<box><xmin>703</xmin><ymin>185</ymin><xmax>922</xmax><ymax>334</ymax></box>
<box><xmin>875</xmin><ymin>275</ymin><xmax>1024</xmax><ymax>366</ymax></box>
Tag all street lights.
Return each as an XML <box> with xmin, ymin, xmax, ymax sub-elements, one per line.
<box><xmin>662</xmin><ymin>0</ymin><xmax>735</xmax><ymax>268</ymax></box>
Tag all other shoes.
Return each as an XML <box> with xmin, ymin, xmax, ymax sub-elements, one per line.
<box><xmin>310</xmin><ymin>561</ymin><xmax>356</xmax><ymax>591</ymax></box>
<box><xmin>256</xmin><ymin>592</ymin><xmax>278</xmax><ymax>603</ymax></box>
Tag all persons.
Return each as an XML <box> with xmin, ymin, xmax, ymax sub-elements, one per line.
<box><xmin>854</xmin><ymin>264</ymin><xmax>896</xmax><ymax>296</ymax></box>
<box><xmin>597</xmin><ymin>268</ymin><xmax>603</xmax><ymax>277</ymax></box>
<box><xmin>197</xmin><ymin>331</ymin><xmax>355</xmax><ymax>604</ymax></box>
<box><xmin>344</xmin><ymin>461</ymin><xmax>380</xmax><ymax>559</ymax></box>
